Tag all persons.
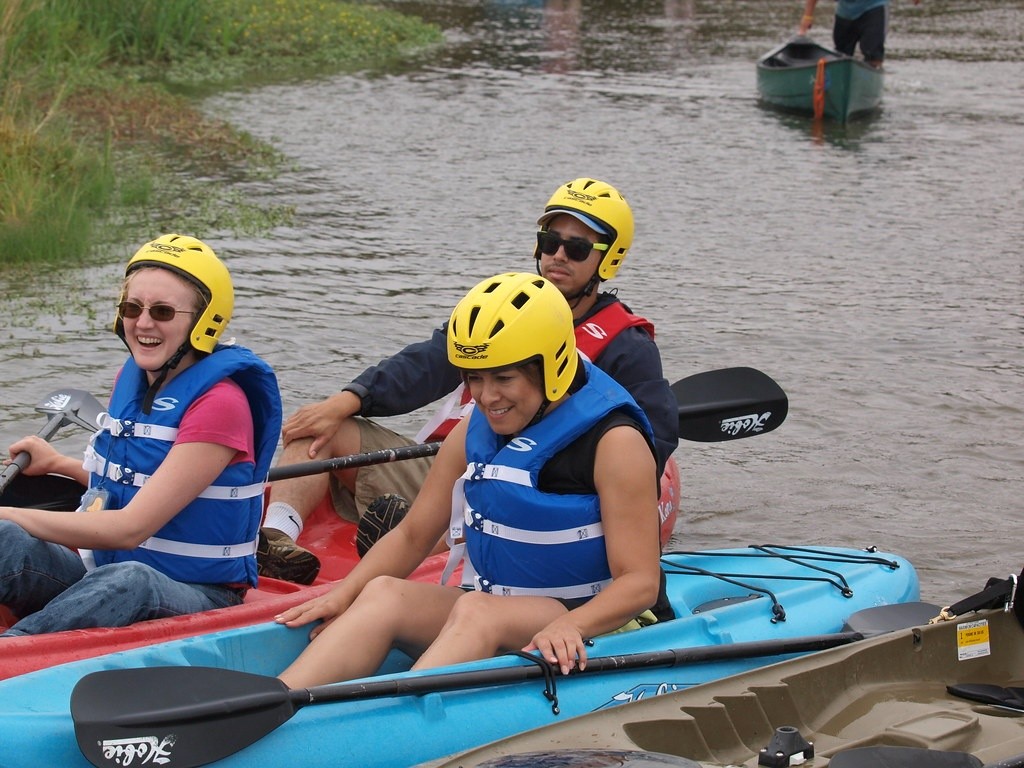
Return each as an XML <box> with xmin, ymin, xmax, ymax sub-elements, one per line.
<box><xmin>799</xmin><ymin>1</ymin><xmax>903</xmax><ymax>68</ymax></box>
<box><xmin>1</xmin><ymin>232</ymin><xmax>283</xmax><ymax>636</ymax></box>
<box><xmin>267</xmin><ymin>274</ymin><xmax>661</xmax><ymax>681</ymax></box>
<box><xmin>255</xmin><ymin>180</ymin><xmax>682</xmax><ymax>582</ymax></box>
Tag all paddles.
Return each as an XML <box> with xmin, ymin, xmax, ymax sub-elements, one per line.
<box><xmin>0</xmin><ymin>388</ymin><xmax>108</xmax><ymax>496</ymax></box>
<box><xmin>264</xmin><ymin>367</ymin><xmax>790</xmax><ymax>485</ymax></box>
<box><xmin>69</xmin><ymin>600</ymin><xmax>943</xmax><ymax>767</ymax></box>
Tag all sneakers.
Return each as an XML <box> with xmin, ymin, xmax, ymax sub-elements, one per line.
<box><xmin>355</xmin><ymin>493</ymin><xmax>409</xmax><ymax>562</ymax></box>
<box><xmin>256</xmin><ymin>527</ymin><xmax>319</xmax><ymax>586</ymax></box>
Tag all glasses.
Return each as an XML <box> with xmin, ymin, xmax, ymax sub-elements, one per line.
<box><xmin>535</xmin><ymin>231</ymin><xmax>610</xmax><ymax>263</ymax></box>
<box><xmin>115</xmin><ymin>301</ymin><xmax>195</xmax><ymax>322</ymax></box>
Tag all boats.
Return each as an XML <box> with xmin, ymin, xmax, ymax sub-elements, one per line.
<box><xmin>0</xmin><ymin>546</ymin><xmax>920</xmax><ymax>766</ymax></box>
<box><xmin>1</xmin><ymin>421</ymin><xmax>679</xmax><ymax>688</ymax></box>
<box><xmin>417</xmin><ymin>566</ymin><xmax>1021</xmax><ymax>765</ymax></box>
<box><xmin>755</xmin><ymin>30</ymin><xmax>883</xmax><ymax>127</ymax></box>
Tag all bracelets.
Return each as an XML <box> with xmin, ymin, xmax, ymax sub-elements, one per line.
<box><xmin>802</xmin><ymin>13</ymin><xmax>813</xmax><ymax>21</ymax></box>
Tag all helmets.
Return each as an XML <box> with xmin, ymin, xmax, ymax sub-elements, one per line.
<box><xmin>111</xmin><ymin>233</ymin><xmax>234</xmax><ymax>355</ymax></box>
<box><xmin>531</xmin><ymin>177</ymin><xmax>634</xmax><ymax>281</ymax></box>
<box><xmin>446</xmin><ymin>273</ymin><xmax>578</xmax><ymax>402</ymax></box>
